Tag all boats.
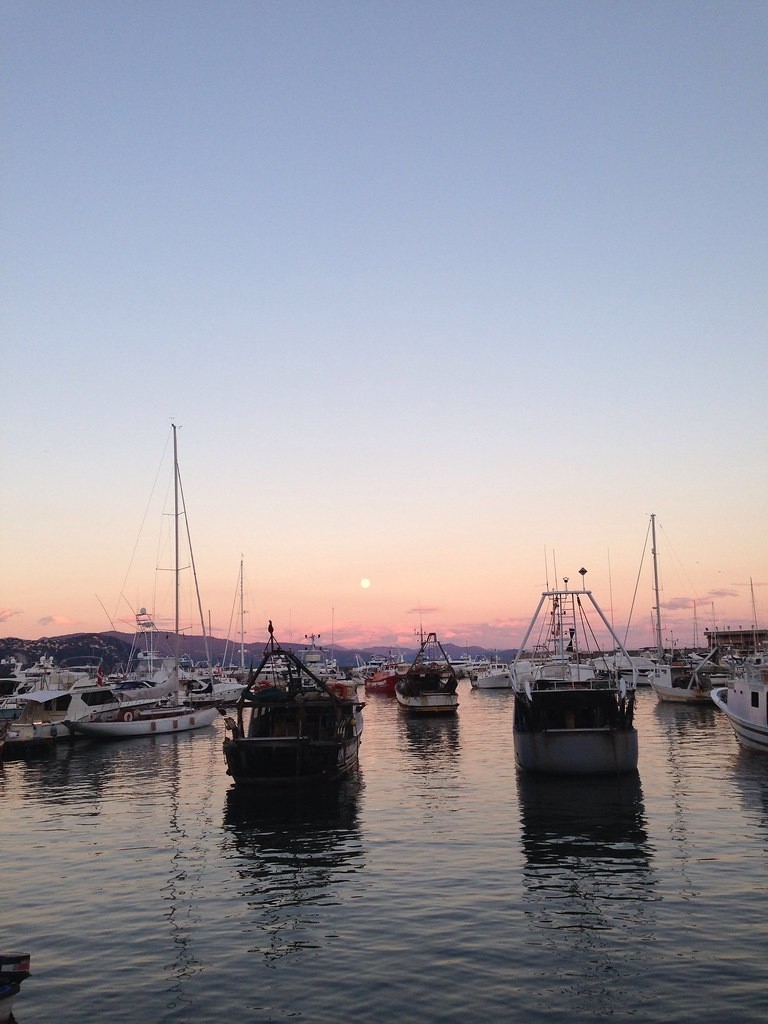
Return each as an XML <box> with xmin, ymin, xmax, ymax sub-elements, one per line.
<box><xmin>710</xmin><ymin>652</ymin><xmax>768</xmax><ymax>749</ymax></box>
<box><xmin>0</xmin><ymin>650</ymin><xmax>768</xmax><ymax>748</ymax></box>
<box><xmin>469</xmin><ymin>662</ymin><xmax>511</xmax><ymax>688</ymax></box>
<box><xmin>646</xmin><ymin>659</ymin><xmax>716</xmax><ymax>701</ymax></box>
<box><xmin>62</xmin><ymin>699</ymin><xmax>222</xmax><ymax>736</ymax></box>
<box><xmin>394</xmin><ymin>633</ymin><xmax>461</xmax><ymax>711</ymax></box>
<box><xmin>215</xmin><ymin>621</ymin><xmax>364</xmax><ymax>781</ymax></box>
<box><xmin>511</xmin><ymin>588</ymin><xmax>638</xmax><ymax>732</ymax></box>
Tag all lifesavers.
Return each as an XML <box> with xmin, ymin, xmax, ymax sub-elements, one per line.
<box><xmin>254</xmin><ymin>679</ymin><xmax>271</xmax><ymax>695</ymax></box>
<box><xmin>331</xmin><ymin>683</ymin><xmax>347</xmax><ymax>700</ymax></box>
